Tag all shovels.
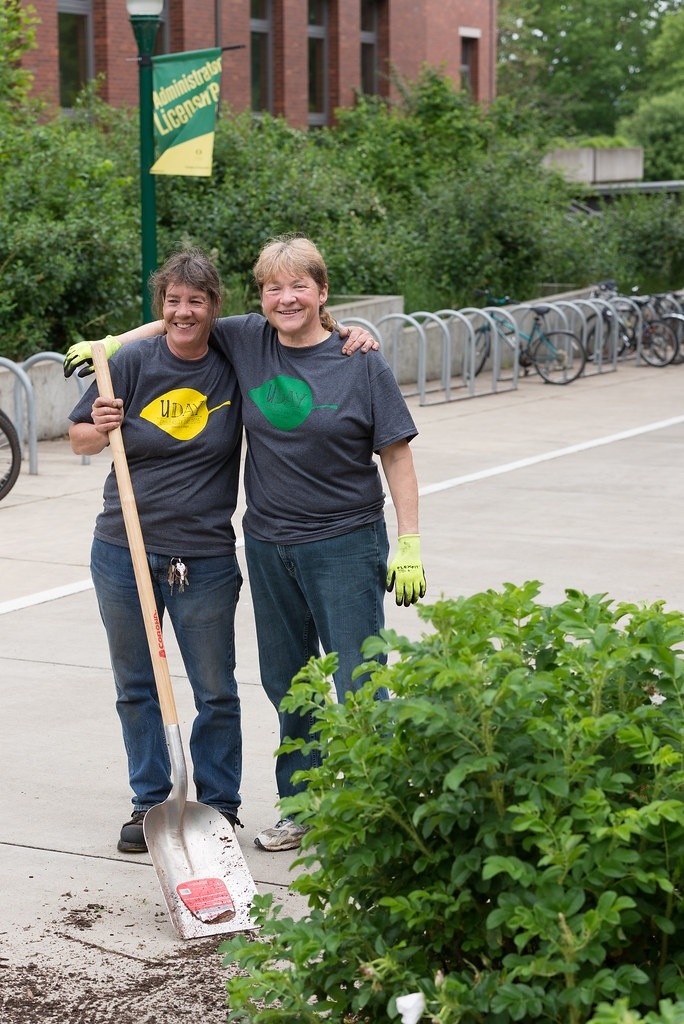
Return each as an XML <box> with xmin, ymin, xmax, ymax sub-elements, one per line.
<box><xmin>90</xmin><ymin>342</ymin><xmax>267</xmax><ymax>943</ymax></box>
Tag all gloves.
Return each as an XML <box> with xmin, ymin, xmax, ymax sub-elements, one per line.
<box><xmin>385</xmin><ymin>534</ymin><xmax>426</xmax><ymax>607</ymax></box>
<box><xmin>63</xmin><ymin>334</ymin><xmax>122</xmax><ymax>379</ymax></box>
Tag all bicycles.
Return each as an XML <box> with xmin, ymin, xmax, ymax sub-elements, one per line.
<box><xmin>579</xmin><ymin>279</ymin><xmax>684</xmax><ymax>369</ymax></box>
<box><xmin>461</xmin><ymin>289</ymin><xmax>587</xmax><ymax>385</ymax></box>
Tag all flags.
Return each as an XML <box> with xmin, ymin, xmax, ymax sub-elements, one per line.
<box><xmin>148</xmin><ymin>47</ymin><xmax>221</xmax><ymax>176</ymax></box>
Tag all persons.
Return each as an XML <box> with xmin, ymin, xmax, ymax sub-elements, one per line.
<box><xmin>64</xmin><ymin>233</ymin><xmax>426</xmax><ymax>853</ymax></box>
<box><xmin>68</xmin><ymin>249</ymin><xmax>380</xmax><ymax>854</ymax></box>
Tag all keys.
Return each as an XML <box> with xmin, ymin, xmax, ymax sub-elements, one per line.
<box><xmin>168</xmin><ymin>557</ymin><xmax>189</xmax><ymax>596</ymax></box>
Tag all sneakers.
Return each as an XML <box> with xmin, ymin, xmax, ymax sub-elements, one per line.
<box><xmin>219</xmin><ymin>811</ymin><xmax>244</xmax><ymax>833</ymax></box>
<box><xmin>118</xmin><ymin>810</ymin><xmax>149</xmax><ymax>852</ymax></box>
<box><xmin>253</xmin><ymin>816</ymin><xmax>316</xmax><ymax>852</ymax></box>
